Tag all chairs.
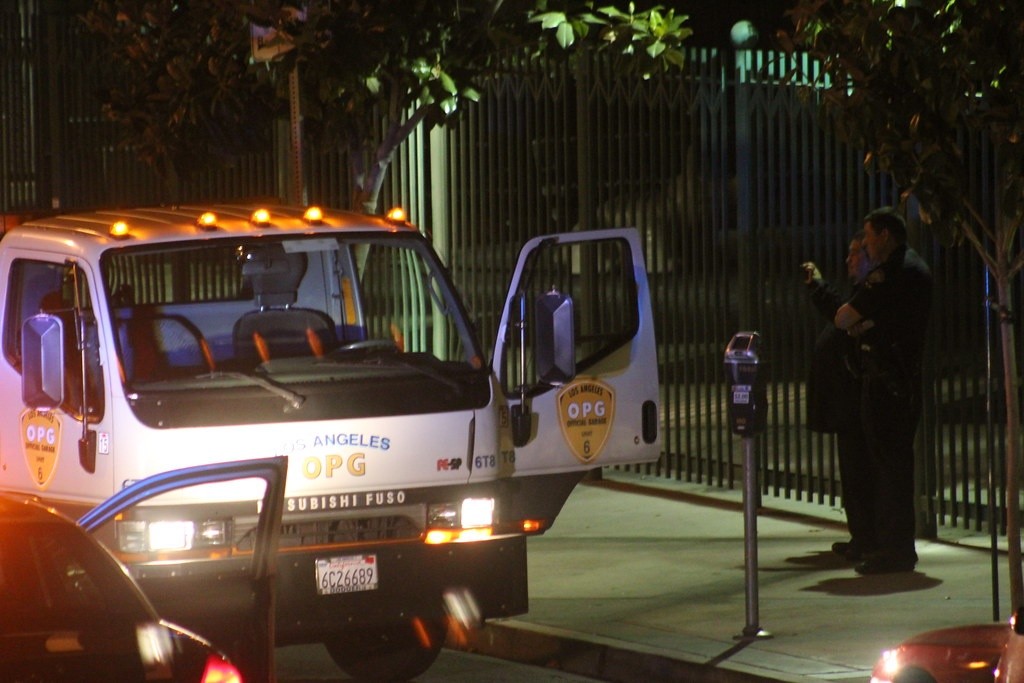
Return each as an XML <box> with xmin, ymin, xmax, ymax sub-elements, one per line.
<box><xmin>233</xmin><ymin>245</ymin><xmax>337</xmax><ymax>376</ymax></box>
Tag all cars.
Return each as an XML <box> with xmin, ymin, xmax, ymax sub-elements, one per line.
<box><xmin>0</xmin><ymin>454</ymin><xmax>289</xmax><ymax>682</ymax></box>
<box><xmin>867</xmin><ymin>604</ymin><xmax>1023</xmax><ymax>682</ymax></box>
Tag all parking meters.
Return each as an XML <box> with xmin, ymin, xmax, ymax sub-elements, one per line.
<box><xmin>721</xmin><ymin>329</ymin><xmax>777</xmax><ymax>640</ymax></box>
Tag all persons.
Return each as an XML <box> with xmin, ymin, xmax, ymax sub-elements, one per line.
<box><xmin>802</xmin><ymin>207</ymin><xmax>930</xmax><ymax>575</ymax></box>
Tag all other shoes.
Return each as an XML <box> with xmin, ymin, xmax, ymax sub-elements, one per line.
<box><xmin>855</xmin><ymin>551</ymin><xmax>918</xmax><ymax>575</ymax></box>
<box><xmin>832</xmin><ymin>541</ymin><xmax>882</xmax><ymax>560</ymax></box>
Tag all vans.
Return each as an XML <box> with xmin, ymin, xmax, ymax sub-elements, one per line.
<box><xmin>0</xmin><ymin>200</ymin><xmax>665</xmax><ymax>682</ymax></box>
